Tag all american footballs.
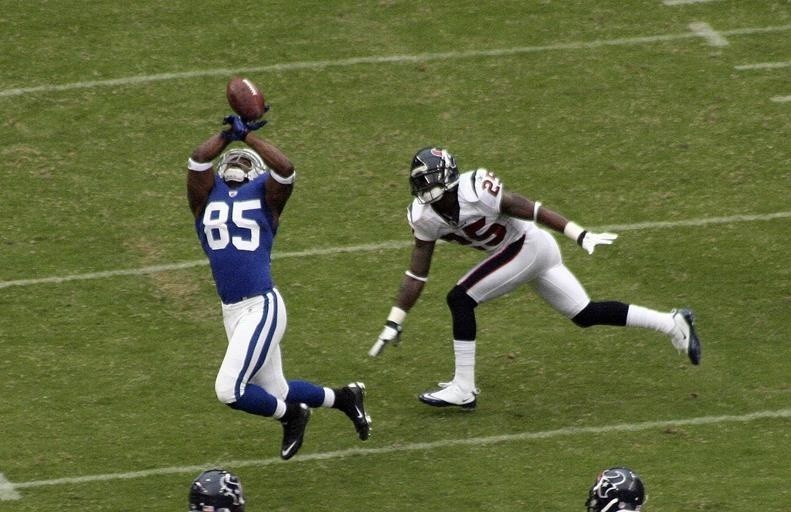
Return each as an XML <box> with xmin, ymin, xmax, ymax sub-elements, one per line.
<box><xmin>227</xmin><ymin>77</ymin><xmax>264</xmax><ymax>116</ymax></box>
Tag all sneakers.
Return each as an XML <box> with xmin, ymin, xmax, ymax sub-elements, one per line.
<box><xmin>418</xmin><ymin>379</ymin><xmax>481</xmax><ymax>409</ymax></box>
<box><xmin>671</xmin><ymin>308</ymin><xmax>701</xmax><ymax>368</ymax></box>
<box><xmin>280</xmin><ymin>402</ymin><xmax>311</xmax><ymax>460</ymax></box>
<box><xmin>343</xmin><ymin>382</ymin><xmax>371</xmax><ymax>442</ymax></box>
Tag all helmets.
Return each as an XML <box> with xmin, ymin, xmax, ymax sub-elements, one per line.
<box><xmin>408</xmin><ymin>146</ymin><xmax>459</xmax><ymax>204</ymax></box>
<box><xmin>216</xmin><ymin>147</ymin><xmax>266</xmax><ymax>181</ymax></box>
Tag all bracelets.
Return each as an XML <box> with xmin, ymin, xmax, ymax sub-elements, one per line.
<box><xmin>563</xmin><ymin>221</ymin><xmax>584</xmax><ymax>242</ymax></box>
<box><xmin>387</xmin><ymin>306</ymin><xmax>407</xmax><ymax>325</ymax></box>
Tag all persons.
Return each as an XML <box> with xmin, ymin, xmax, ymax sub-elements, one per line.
<box><xmin>187</xmin><ymin>103</ymin><xmax>370</xmax><ymax>460</ymax></box>
<box><xmin>370</xmin><ymin>148</ymin><xmax>703</xmax><ymax>412</ymax></box>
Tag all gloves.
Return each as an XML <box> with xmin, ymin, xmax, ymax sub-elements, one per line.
<box><xmin>368</xmin><ymin>307</ymin><xmax>406</xmax><ymax>359</ymax></box>
<box><xmin>563</xmin><ymin>220</ymin><xmax>620</xmax><ymax>256</ymax></box>
<box><xmin>240</xmin><ymin>106</ymin><xmax>270</xmax><ymax>131</ymax></box>
<box><xmin>222</xmin><ymin>113</ymin><xmax>249</xmax><ymax>142</ymax></box>
